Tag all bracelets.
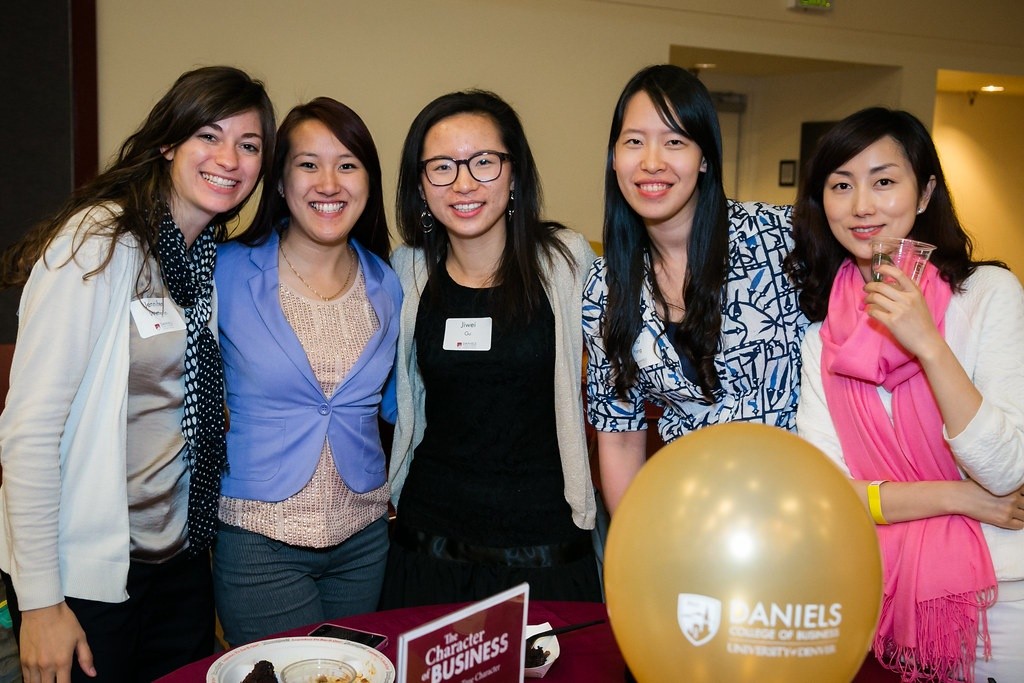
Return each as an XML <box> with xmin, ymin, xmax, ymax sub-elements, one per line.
<box><xmin>867</xmin><ymin>480</ymin><xmax>890</xmax><ymax>525</ymax></box>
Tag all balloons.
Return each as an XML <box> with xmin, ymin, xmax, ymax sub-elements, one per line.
<box><xmin>603</xmin><ymin>422</ymin><xmax>884</xmax><ymax>683</ymax></box>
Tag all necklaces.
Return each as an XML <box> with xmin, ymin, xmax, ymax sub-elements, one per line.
<box><xmin>279</xmin><ymin>240</ymin><xmax>354</xmax><ymax>301</ymax></box>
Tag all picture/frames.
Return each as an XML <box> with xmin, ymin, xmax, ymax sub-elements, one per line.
<box><xmin>779</xmin><ymin>160</ymin><xmax>797</xmax><ymax>187</ymax></box>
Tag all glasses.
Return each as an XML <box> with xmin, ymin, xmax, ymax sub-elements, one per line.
<box><xmin>415</xmin><ymin>149</ymin><xmax>511</xmax><ymax>187</ymax></box>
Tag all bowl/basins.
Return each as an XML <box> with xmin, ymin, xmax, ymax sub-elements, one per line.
<box><xmin>206</xmin><ymin>636</ymin><xmax>396</xmax><ymax>683</ymax></box>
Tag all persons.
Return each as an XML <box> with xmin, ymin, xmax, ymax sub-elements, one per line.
<box><xmin>214</xmin><ymin>97</ymin><xmax>403</xmax><ymax>647</ymax></box>
<box><xmin>582</xmin><ymin>65</ymin><xmax>813</xmax><ymax>514</ymax></box>
<box><xmin>377</xmin><ymin>92</ymin><xmax>603</xmax><ymax>612</ymax></box>
<box><xmin>0</xmin><ymin>66</ymin><xmax>276</xmax><ymax>683</ymax></box>
<box><xmin>781</xmin><ymin>106</ymin><xmax>1024</xmax><ymax>683</ymax></box>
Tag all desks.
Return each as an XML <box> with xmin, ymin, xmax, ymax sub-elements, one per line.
<box><xmin>158</xmin><ymin>600</ymin><xmax>627</xmax><ymax>683</ymax></box>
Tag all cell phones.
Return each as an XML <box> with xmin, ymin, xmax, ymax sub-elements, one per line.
<box><xmin>307</xmin><ymin>623</ymin><xmax>388</xmax><ymax>651</ymax></box>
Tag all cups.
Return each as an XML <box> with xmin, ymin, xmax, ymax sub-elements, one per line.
<box><xmin>281</xmin><ymin>658</ymin><xmax>357</xmax><ymax>683</ymax></box>
<box><xmin>869</xmin><ymin>234</ymin><xmax>939</xmax><ymax>294</ymax></box>
